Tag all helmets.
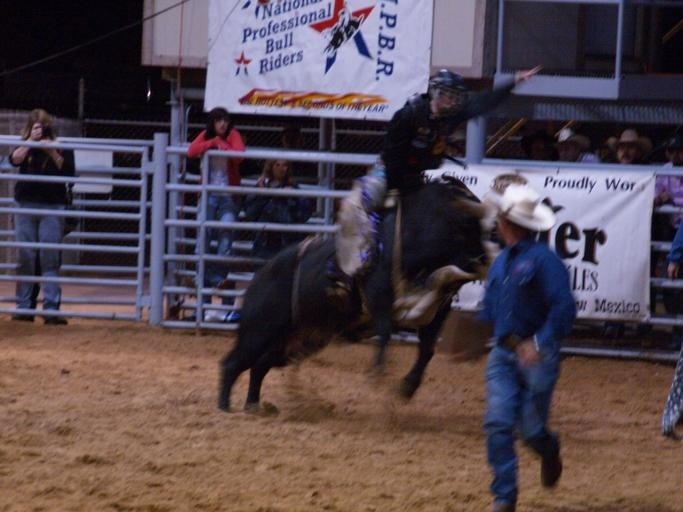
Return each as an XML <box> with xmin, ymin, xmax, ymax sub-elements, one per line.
<box><xmin>427</xmin><ymin>71</ymin><xmax>470</xmax><ymax>118</ymax></box>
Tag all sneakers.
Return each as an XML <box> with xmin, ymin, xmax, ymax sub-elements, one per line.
<box><xmin>541</xmin><ymin>436</ymin><xmax>562</xmax><ymax>487</ymax></box>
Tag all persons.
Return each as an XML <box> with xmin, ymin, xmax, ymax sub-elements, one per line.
<box><xmin>180</xmin><ymin>108</ymin><xmax>682</xmax><ymax>361</ymax></box>
<box><xmin>8</xmin><ymin>108</ymin><xmax>75</xmax><ymax>326</ymax></box>
<box><xmin>458</xmin><ymin>184</ymin><xmax>575</xmax><ymax>510</ymax></box>
<box><xmin>324</xmin><ymin>63</ymin><xmax>543</xmax><ymax>300</ymax></box>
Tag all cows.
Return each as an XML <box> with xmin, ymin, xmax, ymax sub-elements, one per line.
<box><xmin>216</xmin><ymin>174</ymin><xmax>503</xmax><ymax>415</ymax></box>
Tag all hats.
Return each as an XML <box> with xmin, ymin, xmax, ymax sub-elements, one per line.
<box><xmin>521</xmin><ymin>128</ymin><xmax>682</xmax><ymax>161</ymax></box>
<box><xmin>485</xmin><ymin>185</ymin><xmax>557</xmax><ymax>233</ymax></box>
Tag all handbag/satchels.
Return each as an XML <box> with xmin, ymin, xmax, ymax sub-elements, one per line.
<box><xmin>64</xmin><ymin>206</ymin><xmax>78</xmax><ymax>238</ymax></box>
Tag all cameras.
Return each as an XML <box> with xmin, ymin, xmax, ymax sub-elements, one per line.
<box><xmin>41</xmin><ymin>125</ymin><xmax>54</xmax><ymax>141</ymax></box>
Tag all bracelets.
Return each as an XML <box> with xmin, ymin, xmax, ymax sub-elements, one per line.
<box><xmin>54</xmin><ymin>155</ymin><xmax>61</xmax><ymax>164</ymax></box>
